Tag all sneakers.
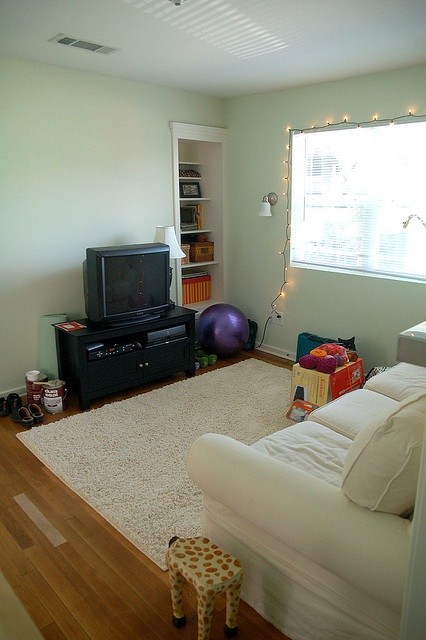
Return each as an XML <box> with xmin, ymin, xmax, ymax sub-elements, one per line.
<box><xmin>6</xmin><ymin>394</ymin><xmax>23</xmax><ymax>415</ymax></box>
<box><xmin>0</xmin><ymin>397</ymin><xmax>10</xmax><ymax>417</ymax></box>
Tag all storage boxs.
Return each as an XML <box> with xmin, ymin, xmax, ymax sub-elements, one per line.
<box><xmin>289</xmin><ymin>357</ymin><xmax>364</xmax><ymax>407</ymax></box>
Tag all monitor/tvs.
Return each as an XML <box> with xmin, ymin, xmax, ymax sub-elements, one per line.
<box><xmin>82</xmin><ymin>242</ymin><xmax>174</xmax><ymax>329</ymax></box>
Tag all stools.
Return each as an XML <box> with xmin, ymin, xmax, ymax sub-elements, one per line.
<box><xmin>166</xmin><ymin>535</ymin><xmax>247</xmax><ymax>640</ymax></box>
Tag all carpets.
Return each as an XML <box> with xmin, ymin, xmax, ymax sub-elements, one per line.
<box><xmin>16</xmin><ymin>355</ymin><xmax>298</xmax><ymax>573</ymax></box>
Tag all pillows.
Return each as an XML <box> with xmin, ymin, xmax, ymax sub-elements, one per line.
<box><xmin>341</xmin><ymin>390</ymin><xmax>425</xmax><ymax>516</ymax></box>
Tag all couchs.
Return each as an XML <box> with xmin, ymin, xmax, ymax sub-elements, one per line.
<box><xmin>185</xmin><ymin>362</ymin><xmax>426</xmax><ymax>639</ymax></box>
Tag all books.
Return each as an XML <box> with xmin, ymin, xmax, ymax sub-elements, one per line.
<box><xmin>182</xmin><ymin>275</ymin><xmax>211</xmax><ymax>304</ymax></box>
<box><xmin>180</xmin><ymin>204</ymin><xmax>198</xmax><ymax>231</ymax></box>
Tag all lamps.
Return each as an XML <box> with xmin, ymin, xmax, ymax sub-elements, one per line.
<box><xmin>153</xmin><ymin>225</ymin><xmax>187</xmax><ymax>310</ymax></box>
<box><xmin>257</xmin><ymin>192</ymin><xmax>278</xmax><ymax>218</ymax></box>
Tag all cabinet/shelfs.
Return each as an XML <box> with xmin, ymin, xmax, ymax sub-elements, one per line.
<box><xmin>170</xmin><ymin>121</ymin><xmax>229</xmax><ymax>344</ymax></box>
<box><xmin>50</xmin><ymin>305</ymin><xmax>198</xmax><ymax>411</ymax></box>
<box><xmin>396</xmin><ymin>320</ymin><xmax>426</xmax><ymax>365</ymax></box>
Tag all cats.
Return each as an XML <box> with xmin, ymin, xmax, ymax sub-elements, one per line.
<box><xmin>338</xmin><ymin>337</ymin><xmax>356</xmax><ymax>352</ymax></box>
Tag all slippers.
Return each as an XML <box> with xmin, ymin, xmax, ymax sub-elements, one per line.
<box><xmin>24</xmin><ymin>403</ymin><xmax>44</xmax><ymax>424</ymax></box>
<box><xmin>10</xmin><ymin>407</ymin><xmax>33</xmax><ymax>428</ymax></box>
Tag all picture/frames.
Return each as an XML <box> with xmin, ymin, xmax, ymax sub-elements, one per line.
<box><xmin>179</xmin><ymin>181</ymin><xmax>201</xmax><ymax>198</ymax></box>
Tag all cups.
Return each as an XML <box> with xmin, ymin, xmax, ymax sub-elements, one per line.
<box><xmin>26</xmin><ymin>370</ymin><xmax>39</xmax><ymax>381</ymax></box>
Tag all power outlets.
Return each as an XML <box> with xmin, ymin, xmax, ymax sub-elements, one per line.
<box><xmin>273</xmin><ymin>311</ymin><xmax>286</xmax><ymax>327</ymax></box>
